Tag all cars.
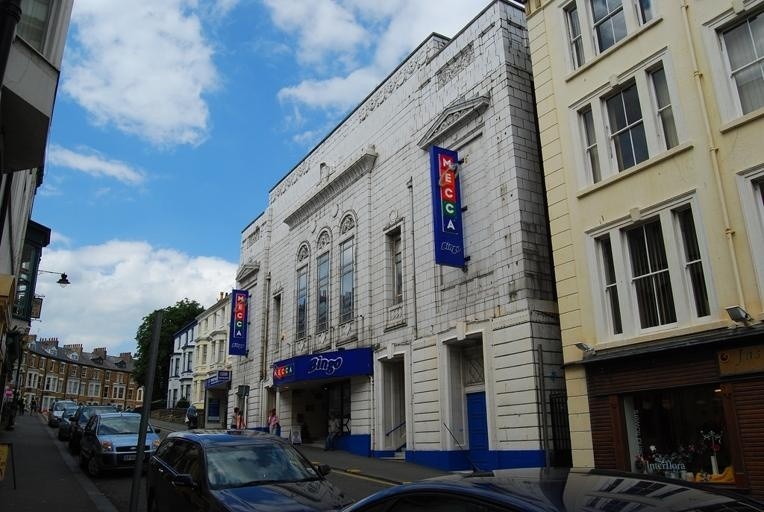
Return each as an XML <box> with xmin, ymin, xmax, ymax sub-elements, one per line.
<box><xmin>79</xmin><ymin>412</ymin><xmax>162</xmax><ymax>476</ymax></box>
<box><xmin>186</xmin><ymin>402</ymin><xmax>204</xmax><ymax>427</ymax></box>
<box><xmin>125</xmin><ymin>409</ymin><xmax>134</xmax><ymax>412</ymax></box>
<box><xmin>337</xmin><ymin>422</ymin><xmax>763</xmax><ymax>512</ymax></box>
<box><xmin>57</xmin><ymin>406</ymin><xmax>79</xmax><ymax>440</ymax></box>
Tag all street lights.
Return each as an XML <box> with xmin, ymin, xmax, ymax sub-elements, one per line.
<box><xmin>36</xmin><ymin>265</ymin><xmax>72</xmax><ymax>290</ymax></box>
<box><xmin>112</xmin><ymin>382</ymin><xmax>126</xmax><ymax>411</ymax></box>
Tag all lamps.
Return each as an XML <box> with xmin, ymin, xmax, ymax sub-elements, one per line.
<box><xmin>725</xmin><ymin>306</ymin><xmax>753</xmax><ymax>327</ymax></box>
<box><xmin>573</xmin><ymin>342</ymin><xmax>590</xmax><ymax>352</ymax></box>
<box><xmin>36</xmin><ymin>269</ymin><xmax>71</xmax><ymax>289</ymax></box>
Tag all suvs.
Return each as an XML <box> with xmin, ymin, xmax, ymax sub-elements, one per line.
<box><xmin>142</xmin><ymin>429</ymin><xmax>355</xmax><ymax>512</ymax></box>
<box><xmin>68</xmin><ymin>404</ymin><xmax>117</xmax><ymax>451</ymax></box>
<box><xmin>48</xmin><ymin>399</ymin><xmax>77</xmax><ymax>427</ymax></box>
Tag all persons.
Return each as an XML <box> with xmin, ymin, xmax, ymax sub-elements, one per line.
<box><xmin>230</xmin><ymin>407</ymin><xmax>247</xmax><ymax>429</ymax></box>
<box><xmin>20</xmin><ymin>397</ymin><xmax>41</xmax><ymax>416</ymax></box>
<box><xmin>187</xmin><ymin>404</ymin><xmax>200</xmax><ymax>428</ymax></box>
<box><xmin>266</xmin><ymin>408</ymin><xmax>280</xmax><ymax>435</ymax></box>
<box><xmin>323</xmin><ymin>409</ymin><xmax>340</xmax><ymax>452</ymax></box>
